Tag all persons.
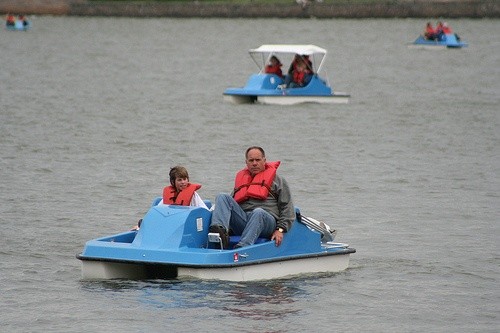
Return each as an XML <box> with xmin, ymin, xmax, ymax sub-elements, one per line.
<box><xmin>6</xmin><ymin>12</ymin><xmax>27</xmax><ymax>26</ymax></box>
<box><xmin>209</xmin><ymin>146</ymin><xmax>296</xmax><ymax>247</ymax></box>
<box><xmin>425</xmin><ymin>21</ymin><xmax>451</xmax><ymax>41</ymax></box>
<box><xmin>130</xmin><ymin>166</ymin><xmax>210</xmax><ymax>231</ymax></box>
<box><xmin>264</xmin><ymin>54</ymin><xmax>313</xmax><ymax>88</ymax></box>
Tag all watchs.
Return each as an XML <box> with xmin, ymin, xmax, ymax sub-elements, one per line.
<box><xmin>274</xmin><ymin>228</ymin><xmax>285</xmax><ymax>233</ymax></box>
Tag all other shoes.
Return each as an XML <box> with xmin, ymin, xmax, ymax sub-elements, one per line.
<box><xmin>234</xmin><ymin>245</ymin><xmax>242</xmax><ymax>249</ymax></box>
<box><xmin>209</xmin><ymin>224</ymin><xmax>229</xmax><ymax>249</ymax></box>
<box><xmin>138</xmin><ymin>218</ymin><xmax>143</xmax><ymax>228</ymax></box>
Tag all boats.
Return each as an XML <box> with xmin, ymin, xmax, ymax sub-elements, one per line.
<box><xmin>6</xmin><ymin>18</ymin><xmax>32</xmax><ymax>32</ymax></box>
<box><xmin>222</xmin><ymin>44</ymin><xmax>351</xmax><ymax>105</ymax></box>
<box><xmin>76</xmin><ymin>198</ymin><xmax>356</xmax><ymax>280</ymax></box>
<box><xmin>407</xmin><ymin>32</ymin><xmax>467</xmax><ymax>47</ymax></box>
